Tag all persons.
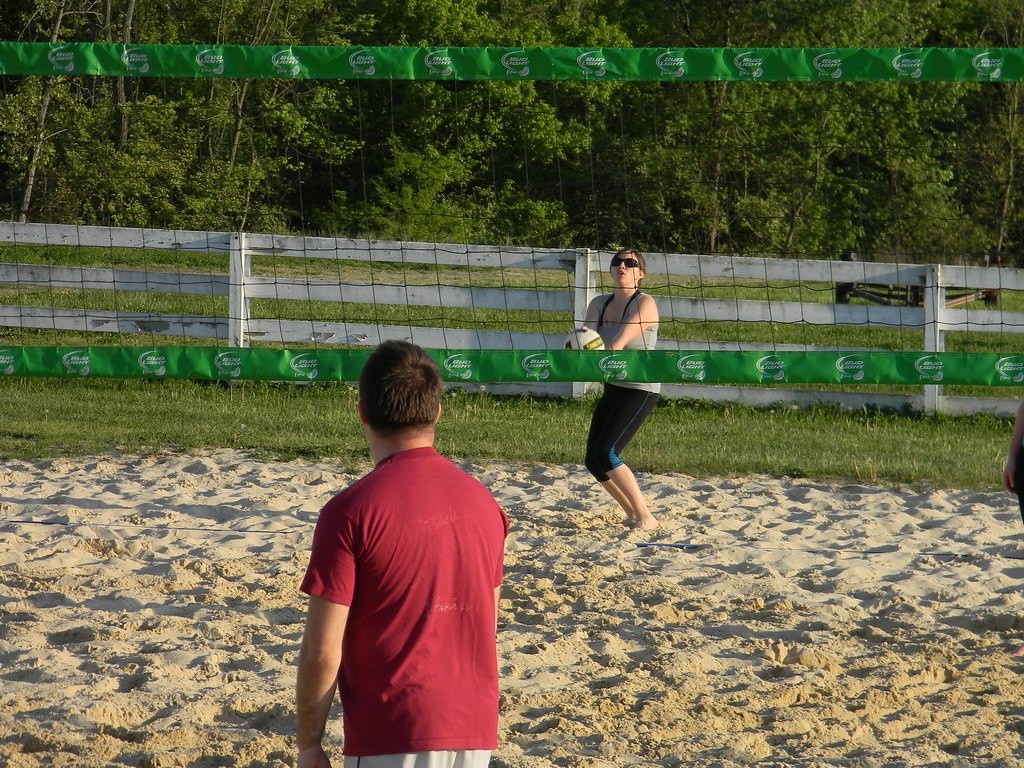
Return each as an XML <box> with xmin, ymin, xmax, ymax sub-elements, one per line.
<box><xmin>296</xmin><ymin>340</ymin><xmax>511</xmax><ymax>768</ymax></box>
<box><xmin>1002</xmin><ymin>397</ymin><xmax>1024</xmax><ymax>522</ymax></box>
<box><xmin>584</xmin><ymin>249</ymin><xmax>663</xmax><ymax>530</ymax></box>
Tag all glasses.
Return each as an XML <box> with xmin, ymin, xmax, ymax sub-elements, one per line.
<box><xmin>612</xmin><ymin>258</ymin><xmax>641</xmax><ymax>268</ymax></box>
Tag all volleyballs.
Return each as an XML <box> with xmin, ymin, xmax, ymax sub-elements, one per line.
<box><xmin>563</xmin><ymin>329</ymin><xmax>605</xmax><ymax>350</ymax></box>
